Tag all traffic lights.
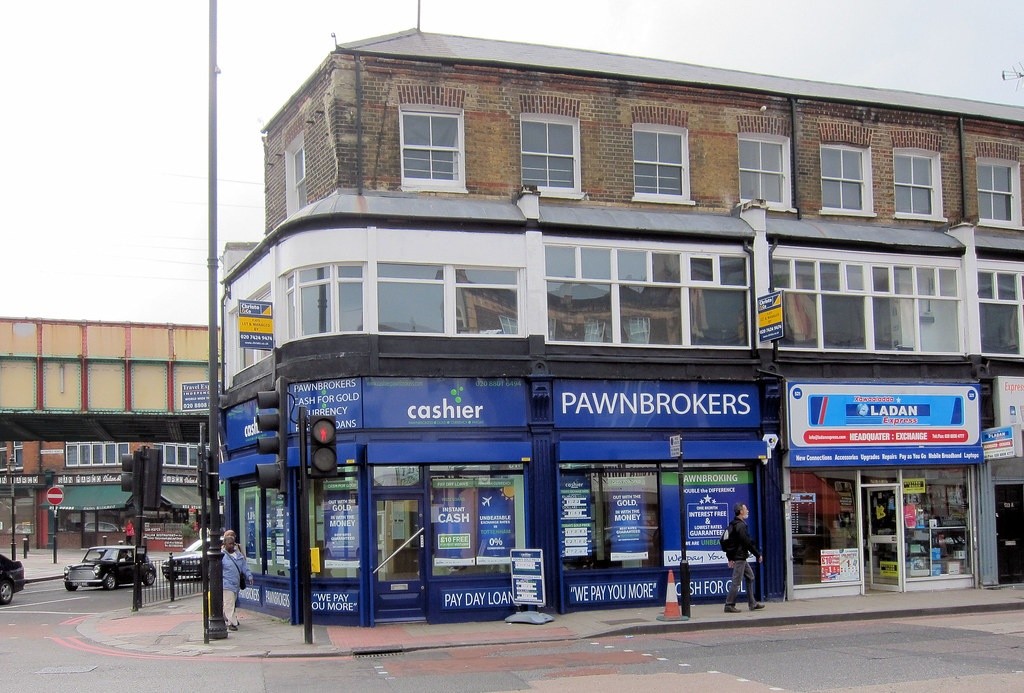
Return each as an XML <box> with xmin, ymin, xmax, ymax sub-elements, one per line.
<box><xmin>120</xmin><ymin>453</ymin><xmax>135</xmax><ymax>493</ymax></box>
<box><xmin>252</xmin><ymin>375</ymin><xmax>287</xmax><ymax>495</ymax></box>
<box><xmin>309</xmin><ymin>415</ymin><xmax>338</xmax><ymax>479</ymax></box>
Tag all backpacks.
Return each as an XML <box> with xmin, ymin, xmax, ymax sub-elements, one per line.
<box><xmin>719</xmin><ymin>520</ymin><xmax>747</xmax><ymax>552</ymax></box>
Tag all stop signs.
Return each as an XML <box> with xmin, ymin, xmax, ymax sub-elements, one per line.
<box><xmin>46</xmin><ymin>487</ymin><xmax>65</xmax><ymax>506</ymax></box>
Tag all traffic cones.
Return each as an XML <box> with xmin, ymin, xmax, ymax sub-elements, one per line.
<box><xmin>654</xmin><ymin>569</ymin><xmax>689</xmax><ymax>622</ymax></box>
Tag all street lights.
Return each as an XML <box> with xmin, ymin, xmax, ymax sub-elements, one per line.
<box><xmin>5</xmin><ymin>450</ymin><xmax>19</xmax><ymax>560</ymax></box>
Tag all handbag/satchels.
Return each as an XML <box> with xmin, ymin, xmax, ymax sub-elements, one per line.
<box><xmin>240</xmin><ymin>571</ymin><xmax>246</xmax><ymax>590</ymax></box>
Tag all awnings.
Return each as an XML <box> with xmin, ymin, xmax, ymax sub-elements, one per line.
<box><xmin>38</xmin><ymin>485</ymin><xmax>133</xmax><ymax>511</ymax></box>
<box><xmin>160</xmin><ymin>485</ymin><xmax>206</xmax><ymax>506</ymax></box>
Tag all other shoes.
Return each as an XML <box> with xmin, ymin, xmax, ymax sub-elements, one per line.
<box><xmin>724</xmin><ymin>605</ymin><xmax>741</xmax><ymax>612</ymax></box>
<box><xmin>748</xmin><ymin>602</ymin><xmax>765</xmax><ymax>610</ymax></box>
<box><xmin>229</xmin><ymin>624</ymin><xmax>238</xmax><ymax>631</ymax></box>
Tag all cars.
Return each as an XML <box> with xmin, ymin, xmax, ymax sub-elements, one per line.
<box><xmin>790</xmin><ymin>517</ymin><xmax>830</xmax><ymax>537</ymax></box>
<box><xmin>160</xmin><ymin>541</ymin><xmax>223</xmax><ymax>581</ymax></box>
<box><xmin>0</xmin><ymin>553</ymin><xmax>25</xmax><ymax>606</ymax></box>
<box><xmin>183</xmin><ymin>536</ymin><xmax>225</xmax><ymax>551</ymax></box>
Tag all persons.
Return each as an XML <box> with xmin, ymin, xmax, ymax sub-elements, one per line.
<box><xmin>125</xmin><ymin>520</ymin><xmax>135</xmax><ymax>545</ymax></box>
<box><xmin>719</xmin><ymin>503</ymin><xmax>765</xmax><ymax>613</ymax></box>
<box><xmin>222</xmin><ymin>530</ymin><xmax>247</xmax><ymax>560</ymax></box>
<box><xmin>221</xmin><ymin>536</ymin><xmax>253</xmax><ymax>631</ymax></box>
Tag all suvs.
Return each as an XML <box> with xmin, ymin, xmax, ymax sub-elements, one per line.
<box><xmin>61</xmin><ymin>545</ymin><xmax>157</xmax><ymax>592</ymax></box>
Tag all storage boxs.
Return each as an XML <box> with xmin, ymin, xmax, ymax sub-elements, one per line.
<box><xmin>953</xmin><ymin>551</ymin><xmax>966</xmax><ymax>559</ymax></box>
